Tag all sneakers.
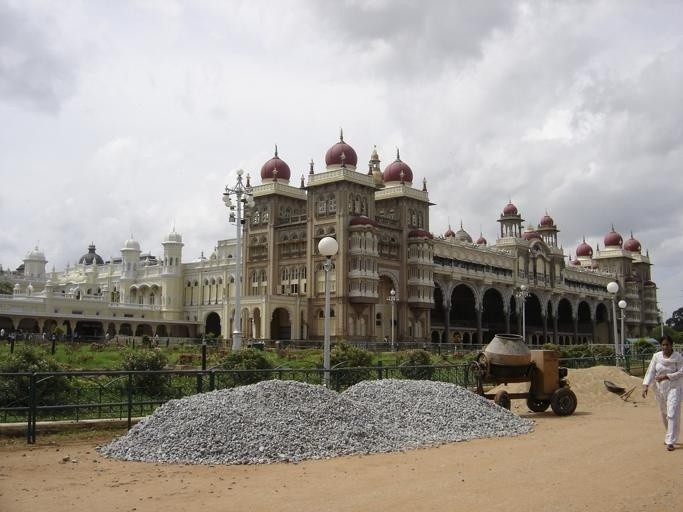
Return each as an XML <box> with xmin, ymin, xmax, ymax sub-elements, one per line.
<box><xmin>664</xmin><ymin>441</ymin><xmax>674</xmax><ymax>451</ymax></box>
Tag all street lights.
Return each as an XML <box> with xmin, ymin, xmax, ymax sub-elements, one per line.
<box><xmin>606</xmin><ymin>281</ymin><xmax>620</xmax><ymax>366</ymax></box>
<box><xmin>389</xmin><ymin>288</ymin><xmax>395</xmax><ymax>352</ymax></box>
<box><xmin>617</xmin><ymin>299</ymin><xmax>626</xmax><ymax>361</ymax></box>
<box><xmin>514</xmin><ymin>284</ymin><xmax>532</xmax><ymax>343</ymax></box>
<box><xmin>316</xmin><ymin>236</ymin><xmax>339</xmax><ymax>388</ymax></box>
<box><xmin>221</xmin><ymin>168</ymin><xmax>256</xmax><ymax>354</ymax></box>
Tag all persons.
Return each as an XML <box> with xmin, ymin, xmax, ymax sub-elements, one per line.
<box><xmin>640</xmin><ymin>335</ymin><xmax>682</xmax><ymax>451</ymax></box>
